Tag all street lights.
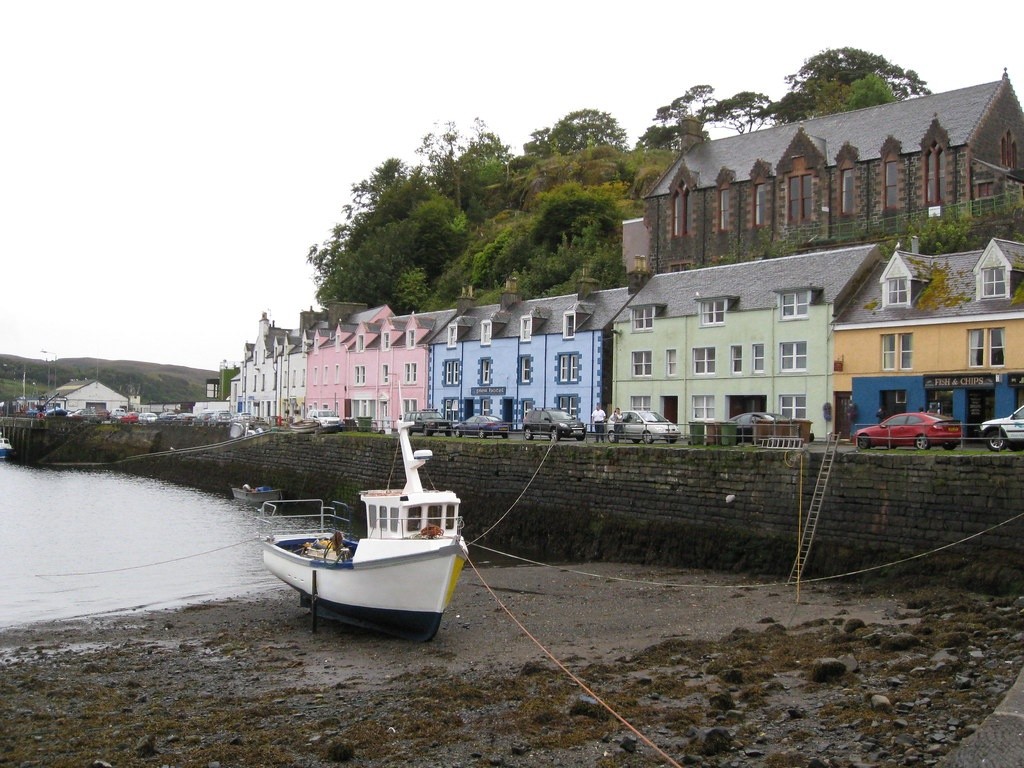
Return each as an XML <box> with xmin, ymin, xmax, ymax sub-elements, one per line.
<box><xmin>42</xmin><ymin>350</ymin><xmax>57</xmax><ymax>416</ymax></box>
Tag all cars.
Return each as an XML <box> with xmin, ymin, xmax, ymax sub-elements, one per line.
<box><xmin>727</xmin><ymin>411</ymin><xmax>788</xmax><ymax>445</ymax></box>
<box><xmin>25</xmin><ymin>406</ymin><xmax>235</xmax><ymax>428</ymax></box>
<box><xmin>234</xmin><ymin>411</ymin><xmax>256</xmax><ymax>430</ymax></box>
<box><xmin>852</xmin><ymin>411</ymin><xmax>964</xmax><ymax>451</ymax></box>
<box><xmin>606</xmin><ymin>410</ymin><xmax>681</xmax><ymax>444</ymax></box>
<box><xmin>453</xmin><ymin>415</ymin><xmax>513</xmax><ymax>440</ymax></box>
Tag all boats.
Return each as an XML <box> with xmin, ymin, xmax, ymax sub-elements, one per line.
<box><xmin>0</xmin><ymin>430</ymin><xmax>17</xmax><ymax>461</ymax></box>
<box><xmin>258</xmin><ymin>377</ymin><xmax>469</xmax><ymax>643</ymax></box>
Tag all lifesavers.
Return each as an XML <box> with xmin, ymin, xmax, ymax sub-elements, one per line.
<box><xmin>36</xmin><ymin>412</ymin><xmax>45</xmax><ymax>419</ymax></box>
<box><xmin>184</xmin><ymin>416</ymin><xmax>190</xmax><ymax>423</ymax></box>
<box><xmin>243</xmin><ymin>484</ymin><xmax>251</xmax><ymax>491</ymax></box>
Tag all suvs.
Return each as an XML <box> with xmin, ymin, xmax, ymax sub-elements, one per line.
<box><xmin>306</xmin><ymin>408</ymin><xmax>342</xmax><ymax>434</ymax></box>
<box><xmin>522</xmin><ymin>407</ymin><xmax>587</xmax><ymax>442</ymax></box>
<box><xmin>404</xmin><ymin>408</ymin><xmax>452</xmax><ymax>437</ymax></box>
<box><xmin>978</xmin><ymin>402</ymin><xmax>1024</xmax><ymax>452</ymax></box>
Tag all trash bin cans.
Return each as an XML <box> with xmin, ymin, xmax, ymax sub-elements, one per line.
<box><xmin>753</xmin><ymin>419</ymin><xmax>774</xmax><ymax>443</ymax></box>
<box><xmin>343</xmin><ymin>419</ymin><xmax>356</xmax><ymax>432</ymax></box>
<box><xmin>270</xmin><ymin>416</ymin><xmax>277</xmax><ymax>427</ymax></box>
<box><xmin>689</xmin><ymin>421</ymin><xmax>706</xmax><ymax>445</ymax></box>
<box><xmin>792</xmin><ymin>419</ymin><xmax>813</xmax><ymax>448</ymax></box>
<box><xmin>721</xmin><ymin>422</ymin><xmax>737</xmax><ymax>446</ymax></box>
<box><xmin>775</xmin><ymin>420</ymin><xmax>790</xmax><ymax>444</ymax></box>
<box><xmin>705</xmin><ymin>423</ymin><xmax>720</xmax><ymax>445</ymax></box>
<box><xmin>277</xmin><ymin>416</ymin><xmax>282</xmax><ymax>426</ymax></box>
<box><xmin>286</xmin><ymin>416</ymin><xmax>293</xmax><ymax>424</ymax></box>
<box><xmin>356</xmin><ymin>416</ymin><xmax>373</xmax><ymax>432</ymax></box>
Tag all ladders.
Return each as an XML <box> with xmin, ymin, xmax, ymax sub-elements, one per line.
<box><xmin>786</xmin><ymin>432</ymin><xmax>844</xmax><ymax>585</ymax></box>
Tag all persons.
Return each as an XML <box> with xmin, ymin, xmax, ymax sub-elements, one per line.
<box><xmin>918</xmin><ymin>404</ymin><xmax>938</xmax><ymax>413</ymax></box>
<box><xmin>876</xmin><ymin>405</ymin><xmax>887</xmax><ymax>423</ymax></box>
<box><xmin>591</xmin><ymin>405</ymin><xmax>607</xmax><ymax>443</ymax></box>
<box><xmin>612</xmin><ymin>408</ymin><xmax>623</xmax><ymax>443</ymax></box>
